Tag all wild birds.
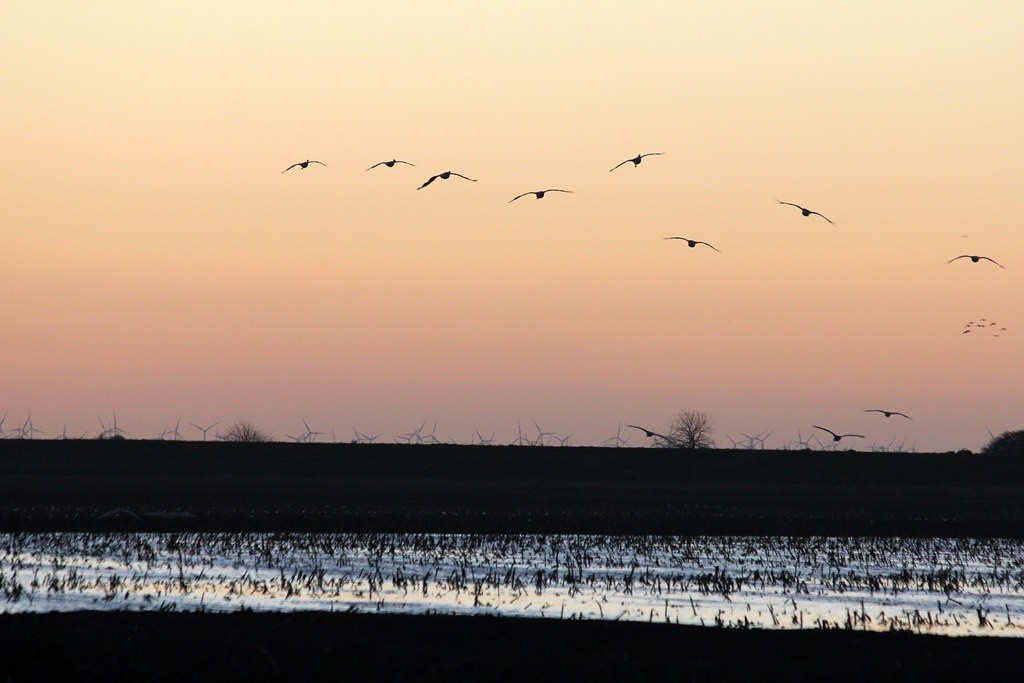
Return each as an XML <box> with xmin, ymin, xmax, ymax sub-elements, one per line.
<box><xmin>281</xmin><ymin>159</ymin><xmax>327</xmax><ymax>175</ymax></box>
<box><xmin>664</xmin><ymin>236</ymin><xmax>723</xmax><ymax>252</ymax></box>
<box><xmin>962</xmin><ymin>318</ymin><xmax>1008</xmax><ymax>338</ymax></box>
<box><xmin>415</xmin><ymin>171</ymin><xmax>478</xmax><ymax>191</ymax></box>
<box><xmin>628</xmin><ymin>424</ymin><xmax>674</xmax><ymax>444</ymax></box>
<box><xmin>364</xmin><ymin>158</ymin><xmax>414</xmax><ymax>172</ymax></box>
<box><xmin>863</xmin><ymin>408</ymin><xmax>913</xmax><ymax>420</ymax></box>
<box><xmin>812</xmin><ymin>424</ymin><xmax>866</xmax><ymax>443</ymax></box>
<box><xmin>776</xmin><ymin>198</ymin><xmax>836</xmax><ymax>226</ymax></box>
<box><xmin>607</xmin><ymin>152</ymin><xmax>666</xmax><ymax>173</ymax></box>
<box><xmin>946</xmin><ymin>254</ymin><xmax>1007</xmax><ymax>270</ymax></box>
<box><xmin>507</xmin><ymin>188</ymin><xmax>574</xmax><ymax>204</ymax></box>
